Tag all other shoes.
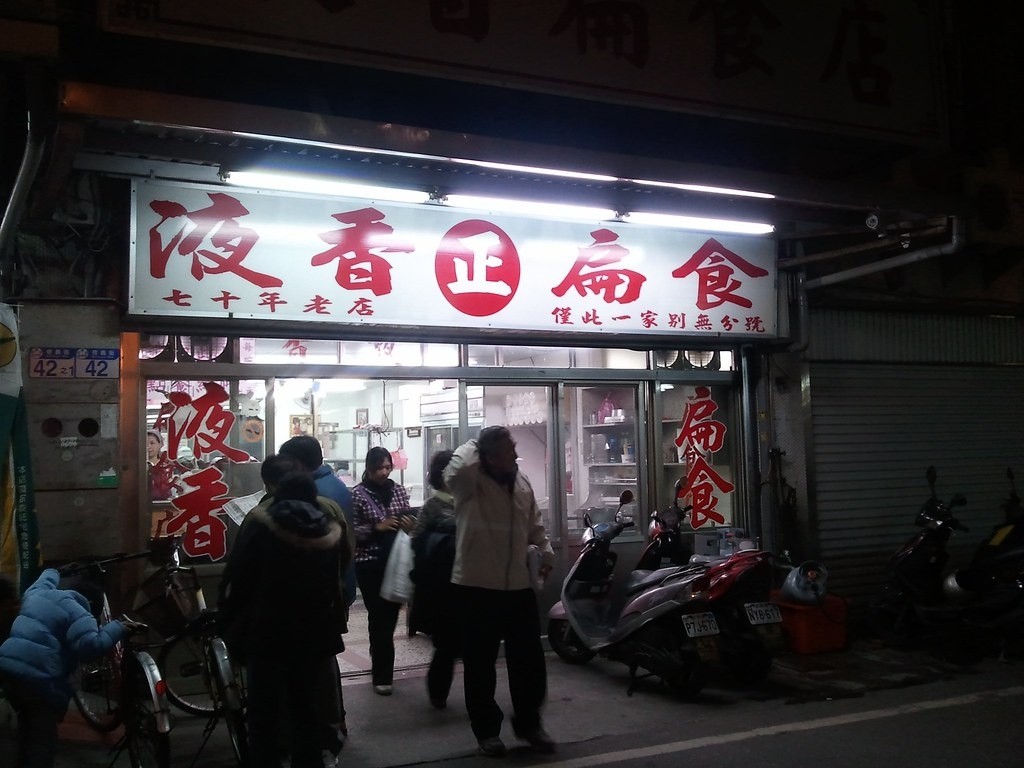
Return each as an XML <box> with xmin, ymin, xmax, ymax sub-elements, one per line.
<box><xmin>430</xmin><ymin>693</ymin><xmax>446</xmax><ymax>709</ymax></box>
<box><xmin>375</xmin><ymin>685</ymin><xmax>393</xmax><ymax>695</ymax></box>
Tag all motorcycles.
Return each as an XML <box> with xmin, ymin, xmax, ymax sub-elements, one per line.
<box><xmin>637</xmin><ymin>476</ymin><xmax>785</xmax><ymax>665</ymax></box>
<box><xmin>547</xmin><ymin>489</ymin><xmax>726</xmax><ymax>688</ymax></box>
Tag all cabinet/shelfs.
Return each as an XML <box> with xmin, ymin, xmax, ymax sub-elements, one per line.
<box><xmin>582</xmin><ymin>416</ymin><xmax>716</xmax><ymax>507</ymax></box>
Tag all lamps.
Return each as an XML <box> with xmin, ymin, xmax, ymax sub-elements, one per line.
<box><xmin>620</xmin><ymin>208</ymin><xmax>776</xmax><ymax>234</ymax></box>
<box><xmin>216</xmin><ymin>167</ymin><xmax>436</xmax><ymax>204</ymax></box>
<box><xmin>435</xmin><ymin>192</ymin><xmax>621</xmax><ymax>221</ymax></box>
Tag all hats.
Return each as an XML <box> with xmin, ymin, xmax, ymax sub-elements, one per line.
<box><xmin>268</xmin><ymin>500</ymin><xmax>329</xmax><ymax>537</ymax></box>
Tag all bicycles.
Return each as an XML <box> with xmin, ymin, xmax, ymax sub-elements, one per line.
<box><xmin>156</xmin><ymin>542</ymin><xmax>265</xmax><ymax>768</ymax></box>
<box><xmin>49</xmin><ymin>548</ymin><xmax>180</xmax><ymax>767</ymax></box>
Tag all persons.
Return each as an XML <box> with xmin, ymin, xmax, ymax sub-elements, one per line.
<box><xmin>441</xmin><ymin>425</ymin><xmax>556</xmax><ymax>759</ymax></box>
<box><xmin>279</xmin><ymin>434</ymin><xmax>353</xmax><ymax>603</ymax></box>
<box><xmin>351</xmin><ymin>446</ymin><xmax>413</xmax><ymax>695</ymax></box>
<box><xmin>218</xmin><ymin>452</ymin><xmax>349</xmax><ymax>768</ymax></box>
<box><xmin>0</xmin><ymin>552</ymin><xmax>138</xmax><ymax>768</ymax></box>
<box><xmin>147</xmin><ymin>429</ymin><xmax>172</xmax><ymax>500</ymax></box>
<box><xmin>409</xmin><ymin>449</ymin><xmax>465</xmax><ymax>711</ymax></box>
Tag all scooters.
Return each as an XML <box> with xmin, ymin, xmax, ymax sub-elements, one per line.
<box><xmin>874</xmin><ymin>465</ymin><xmax>1024</xmax><ymax>660</ymax></box>
<box><xmin>974</xmin><ymin>468</ymin><xmax>1024</xmax><ymax>574</ymax></box>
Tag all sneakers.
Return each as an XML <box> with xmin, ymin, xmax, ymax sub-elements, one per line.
<box><xmin>514</xmin><ymin>725</ymin><xmax>559</xmax><ymax>754</ymax></box>
<box><xmin>477</xmin><ymin>733</ymin><xmax>505</xmax><ymax>755</ymax></box>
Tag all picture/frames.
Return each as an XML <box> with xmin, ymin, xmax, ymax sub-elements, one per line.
<box><xmin>356</xmin><ymin>409</ymin><xmax>368</xmax><ymax>425</ymax></box>
<box><xmin>290</xmin><ymin>414</ymin><xmax>313</xmax><ymax>437</ymax></box>
